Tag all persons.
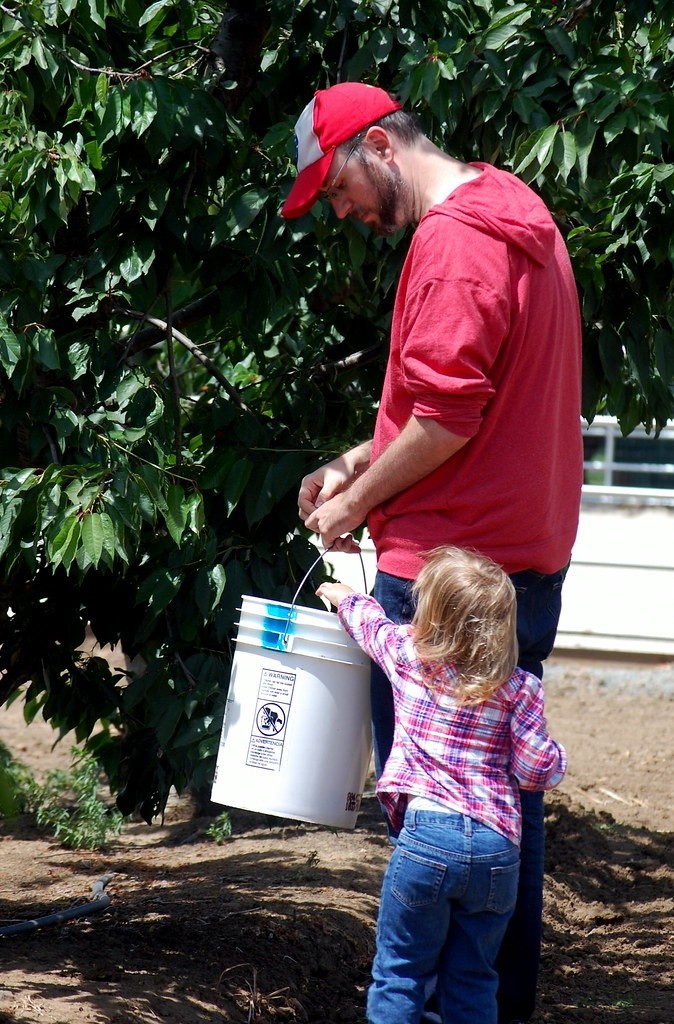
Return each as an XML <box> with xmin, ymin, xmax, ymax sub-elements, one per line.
<box><xmin>315</xmin><ymin>543</ymin><xmax>568</xmax><ymax>1024</ymax></box>
<box><xmin>279</xmin><ymin>82</ymin><xmax>583</xmax><ymax>1023</ymax></box>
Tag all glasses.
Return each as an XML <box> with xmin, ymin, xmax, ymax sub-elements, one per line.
<box><xmin>321</xmin><ymin>134</ymin><xmax>366</xmax><ymax>202</ymax></box>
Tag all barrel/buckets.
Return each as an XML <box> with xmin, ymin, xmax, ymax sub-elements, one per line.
<box><xmin>210</xmin><ymin>547</ymin><xmax>372</xmax><ymax>830</ymax></box>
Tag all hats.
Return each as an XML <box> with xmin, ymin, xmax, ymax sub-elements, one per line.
<box><xmin>280</xmin><ymin>83</ymin><xmax>401</xmax><ymax>220</ymax></box>
